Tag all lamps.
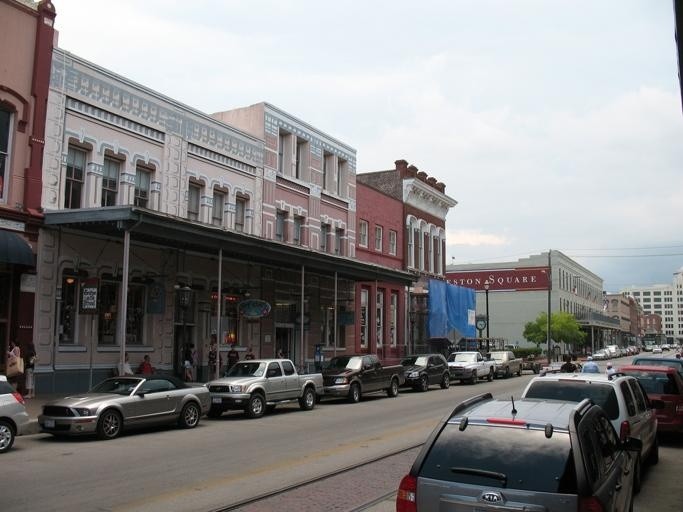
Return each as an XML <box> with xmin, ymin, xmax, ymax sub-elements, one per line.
<box><xmin>176</xmin><ymin>245</ymin><xmax>193</xmax><ymax>313</ymax></box>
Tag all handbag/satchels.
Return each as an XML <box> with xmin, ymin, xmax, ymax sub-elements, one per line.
<box><xmin>6</xmin><ymin>352</ymin><xmax>24</xmax><ymax>378</ymax></box>
<box><xmin>27</xmin><ymin>349</ymin><xmax>39</xmax><ymax>365</ymax></box>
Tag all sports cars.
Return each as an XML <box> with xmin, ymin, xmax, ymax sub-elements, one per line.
<box><xmin>39</xmin><ymin>373</ymin><xmax>211</xmax><ymax>440</ymax></box>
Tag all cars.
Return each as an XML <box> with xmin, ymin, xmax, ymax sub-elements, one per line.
<box><xmin>591</xmin><ymin>340</ymin><xmax>680</xmax><ymax>361</ymax></box>
<box><xmin>0</xmin><ymin>374</ymin><xmax>28</xmax><ymax>451</ymax></box>
<box><xmin>631</xmin><ymin>354</ymin><xmax>682</xmax><ymax>380</ymax></box>
<box><xmin>446</xmin><ymin>343</ymin><xmax>495</xmax><ymax>351</ymax></box>
<box><xmin>503</xmin><ymin>344</ymin><xmax>519</xmax><ymax>349</ymax></box>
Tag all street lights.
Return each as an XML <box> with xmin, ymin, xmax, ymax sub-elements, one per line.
<box><xmin>409</xmin><ymin>310</ymin><xmax>416</xmax><ymax>356</ymax></box>
<box><xmin>540</xmin><ymin>269</ymin><xmax>582</xmax><ymax>364</ymax></box>
<box><xmin>483</xmin><ymin>279</ymin><xmax>491</xmax><ymax>353</ymax></box>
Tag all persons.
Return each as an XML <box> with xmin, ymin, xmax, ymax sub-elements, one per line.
<box><xmin>6</xmin><ymin>339</ymin><xmax>20</xmax><ymax>390</ymax></box>
<box><xmin>560</xmin><ymin>355</ymin><xmax>579</xmax><ymax>373</ymax></box>
<box><xmin>605</xmin><ymin>362</ymin><xmax>616</xmax><ymax>377</ymax></box>
<box><xmin>208</xmin><ymin>343</ymin><xmax>222</xmax><ymax>380</ymax></box>
<box><xmin>23</xmin><ymin>342</ymin><xmax>40</xmax><ymax>399</ymax></box>
<box><xmin>118</xmin><ymin>352</ymin><xmax>133</xmax><ymax>376</ymax></box>
<box><xmin>244</xmin><ymin>347</ymin><xmax>255</xmax><ymax>359</ymax></box>
<box><xmin>189</xmin><ymin>343</ymin><xmax>199</xmax><ymax>381</ymax></box>
<box><xmin>227</xmin><ymin>345</ymin><xmax>239</xmax><ymax>369</ymax></box>
<box><xmin>184</xmin><ymin>343</ymin><xmax>193</xmax><ymax>381</ymax></box>
<box><xmin>581</xmin><ymin>356</ymin><xmax>599</xmax><ymax>373</ymax></box>
<box><xmin>140</xmin><ymin>355</ymin><xmax>152</xmax><ymax>376</ymax></box>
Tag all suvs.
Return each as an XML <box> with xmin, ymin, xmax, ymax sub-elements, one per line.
<box><xmin>394</xmin><ymin>393</ymin><xmax>642</xmax><ymax>510</ymax></box>
<box><xmin>394</xmin><ymin>353</ymin><xmax>450</xmax><ymax>392</ymax></box>
<box><xmin>519</xmin><ymin>372</ymin><xmax>660</xmax><ymax>496</ymax></box>
<box><xmin>618</xmin><ymin>365</ymin><xmax>682</xmax><ymax>434</ymax></box>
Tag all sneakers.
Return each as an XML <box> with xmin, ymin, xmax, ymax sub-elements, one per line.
<box><xmin>22</xmin><ymin>393</ymin><xmax>35</xmax><ymax>399</ymax></box>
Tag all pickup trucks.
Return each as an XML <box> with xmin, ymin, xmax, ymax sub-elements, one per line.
<box><xmin>204</xmin><ymin>358</ymin><xmax>324</xmax><ymax>418</ymax></box>
<box><xmin>485</xmin><ymin>350</ymin><xmax>524</xmax><ymax>378</ymax></box>
<box><xmin>317</xmin><ymin>353</ymin><xmax>402</xmax><ymax>404</ymax></box>
<box><xmin>445</xmin><ymin>352</ymin><xmax>498</xmax><ymax>385</ymax></box>
